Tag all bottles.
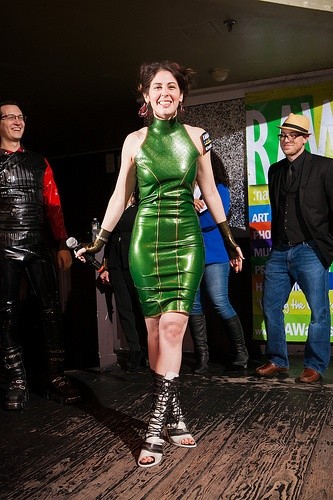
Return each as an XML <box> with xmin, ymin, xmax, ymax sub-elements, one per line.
<box><xmin>91</xmin><ymin>218</ymin><xmax>100</xmax><ymax>242</ymax></box>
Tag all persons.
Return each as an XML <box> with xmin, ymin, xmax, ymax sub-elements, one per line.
<box><xmin>0</xmin><ymin>102</ymin><xmax>84</xmax><ymax>409</ymax></box>
<box><xmin>100</xmin><ymin>194</ymin><xmax>149</xmax><ymax>373</ymax></box>
<box><xmin>74</xmin><ymin>59</ymin><xmax>245</xmax><ymax>469</ymax></box>
<box><xmin>192</xmin><ymin>150</ymin><xmax>248</xmax><ymax>374</ymax></box>
<box><xmin>255</xmin><ymin>110</ymin><xmax>333</xmax><ymax>381</ymax></box>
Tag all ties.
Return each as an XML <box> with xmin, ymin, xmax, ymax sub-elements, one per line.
<box><xmin>286</xmin><ymin>163</ymin><xmax>293</xmax><ymax>191</ymax></box>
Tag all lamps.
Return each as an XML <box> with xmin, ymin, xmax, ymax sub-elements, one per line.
<box><xmin>207</xmin><ymin>68</ymin><xmax>230</xmax><ymax>81</ymax></box>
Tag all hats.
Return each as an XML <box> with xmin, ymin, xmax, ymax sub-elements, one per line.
<box><xmin>277</xmin><ymin>112</ymin><xmax>312</xmax><ymax>135</ymax></box>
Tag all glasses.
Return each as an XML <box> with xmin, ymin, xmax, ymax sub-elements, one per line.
<box><xmin>0</xmin><ymin>114</ymin><xmax>26</xmax><ymax>122</ymax></box>
<box><xmin>277</xmin><ymin>134</ymin><xmax>305</xmax><ymax>140</ymax></box>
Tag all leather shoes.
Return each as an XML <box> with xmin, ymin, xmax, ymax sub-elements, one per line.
<box><xmin>299</xmin><ymin>368</ymin><xmax>322</xmax><ymax>383</ymax></box>
<box><xmin>255</xmin><ymin>361</ymin><xmax>289</xmax><ymax>376</ymax></box>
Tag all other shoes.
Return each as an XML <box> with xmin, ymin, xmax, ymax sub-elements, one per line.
<box><xmin>40</xmin><ymin>372</ymin><xmax>82</xmax><ymax>405</ymax></box>
<box><xmin>2</xmin><ymin>379</ymin><xmax>30</xmax><ymax>410</ymax></box>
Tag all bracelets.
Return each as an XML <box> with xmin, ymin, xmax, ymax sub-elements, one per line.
<box><xmin>96</xmin><ymin>235</ymin><xmax>108</xmax><ymax>242</ymax></box>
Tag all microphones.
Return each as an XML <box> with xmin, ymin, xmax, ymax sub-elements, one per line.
<box><xmin>65</xmin><ymin>236</ymin><xmax>106</xmax><ymax>274</ymax></box>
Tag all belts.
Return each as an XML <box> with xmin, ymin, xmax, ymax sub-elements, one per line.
<box><xmin>287</xmin><ymin>241</ymin><xmax>303</xmax><ymax>247</ymax></box>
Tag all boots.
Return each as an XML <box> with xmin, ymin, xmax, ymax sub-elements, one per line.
<box><xmin>149</xmin><ymin>368</ymin><xmax>198</xmax><ymax>448</ymax></box>
<box><xmin>188</xmin><ymin>314</ymin><xmax>210</xmax><ymax>374</ymax></box>
<box><xmin>137</xmin><ymin>370</ymin><xmax>180</xmax><ymax>467</ymax></box>
<box><xmin>223</xmin><ymin>314</ymin><xmax>250</xmax><ymax>369</ymax></box>
